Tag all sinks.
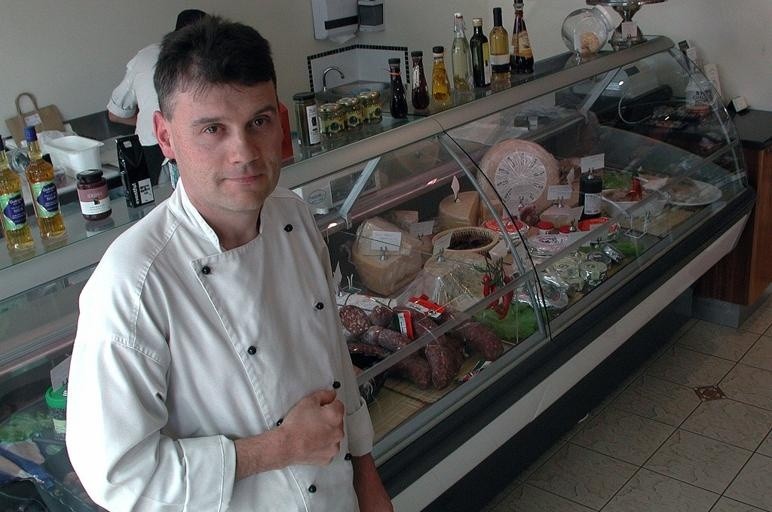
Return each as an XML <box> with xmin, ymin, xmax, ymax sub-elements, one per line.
<box><xmin>329</xmin><ymin>80</ymin><xmax>389</xmax><ymax>99</ymax></box>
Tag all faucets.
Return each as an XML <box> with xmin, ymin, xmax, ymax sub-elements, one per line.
<box><xmin>321</xmin><ymin>66</ymin><xmax>345</xmax><ymax>93</ymax></box>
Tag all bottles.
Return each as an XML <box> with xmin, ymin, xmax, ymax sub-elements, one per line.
<box><xmin>538</xmin><ymin>221</ymin><xmax>570</xmax><ymax>234</ymax></box>
<box><xmin>319</xmin><ymin>90</ymin><xmax>383</xmax><ymax>140</ymax></box>
<box><xmin>676</xmin><ymin>41</ymin><xmax>719</xmax><ymax>110</ymax></box>
<box><xmin>321</xmin><ymin>127</ymin><xmax>374</xmax><ymax>151</ymax></box>
<box><xmin>45</xmin><ymin>386</ymin><xmax>66</xmax><ymax>435</ymax></box>
<box><xmin>387</xmin><ymin>46</ymin><xmax>451</xmax><ymax>118</ymax></box>
<box><xmin>457</xmin><ymin>91</ymin><xmax>491</xmax><ymax>113</ymax></box>
<box><xmin>301</xmin><ymin>147</ymin><xmax>321</xmax><ymax>162</ymax></box>
<box><xmin>580</xmin><ymin>167</ymin><xmax>602</xmax><ymax>218</ymax></box>
<box><xmin>489</xmin><ymin>8</ymin><xmax>510</xmax><ymax>82</ymax></box>
<box><xmin>452</xmin><ymin>11</ymin><xmax>491</xmax><ymax>90</ymax></box>
<box><xmin>1</xmin><ymin>136</ymin><xmax>34</xmax><ymax>253</ymax></box>
<box><xmin>85</xmin><ymin>215</ymin><xmax>115</xmax><ymax>237</ymax></box>
<box><xmin>510</xmin><ymin>0</ymin><xmax>535</xmax><ymax>77</ymax></box>
<box><xmin>491</xmin><ymin>83</ymin><xmax>512</xmax><ymax>93</ymax></box>
<box><xmin>25</xmin><ymin>126</ymin><xmax>65</xmax><ymax>241</ymax></box>
<box><xmin>562</xmin><ymin>4</ymin><xmax>614</xmax><ymax>55</ymax></box>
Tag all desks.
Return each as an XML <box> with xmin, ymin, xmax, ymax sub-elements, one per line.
<box><xmin>612</xmin><ymin>98</ymin><xmax>772</xmax><ymax>328</ymax></box>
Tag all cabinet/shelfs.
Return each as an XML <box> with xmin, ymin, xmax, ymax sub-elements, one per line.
<box><xmin>0</xmin><ymin>37</ymin><xmax>755</xmax><ymax>512</ymax></box>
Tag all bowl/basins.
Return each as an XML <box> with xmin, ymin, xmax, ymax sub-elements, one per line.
<box><xmin>603</xmin><ymin>186</ymin><xmax>666</xmax><ymax>217</ymax></box>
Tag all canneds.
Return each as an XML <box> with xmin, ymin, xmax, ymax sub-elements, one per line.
<box><xmin>318</xmin><ymin>102</ymin><xmax>346</xmax><ymax>140</ymax></box>
<box><xmin>302</xmin><ymin>121</ymin><xmax>305</xmax><ymax>123</ymax></box>
<box><xmin>339</xmin><ymin>96</ymin><xmax>364</xmax><ymax>133</ymax></box>
<box><xmin>361</xmin><ymin>91</ymin><xmax>385</xmax><ymax>126</ymax></box>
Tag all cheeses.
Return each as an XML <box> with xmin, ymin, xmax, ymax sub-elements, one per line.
<box><xmin>351</xmin><ymin>140</ymin><xmax>573</xmax><ymax>307</ymax></box>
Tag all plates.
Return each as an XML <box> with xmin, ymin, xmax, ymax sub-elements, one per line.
<box><xmin>642</xmin><ymin>177</ymin><xmax>722</xmax><ymax>205</ymax></box>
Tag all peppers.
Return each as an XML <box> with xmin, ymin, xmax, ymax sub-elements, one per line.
<box><xmin>481</xmin><ymin>252</ymin><xmax>514</xmax><ymax>320</ymax></box>
<box><xmin>629</xmin><ymin>170</ymin><xmax>642</xmax><ymax>201</ymax></box>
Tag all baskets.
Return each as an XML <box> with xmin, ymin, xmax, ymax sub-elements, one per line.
<box><xmin>431</xmin><ymin>224</ymin><xmax>501</xmax><ymax>261</ymax></box>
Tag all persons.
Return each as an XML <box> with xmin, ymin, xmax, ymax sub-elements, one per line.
<box><xmin>66</xmin><ymin>14</ymin><xmax>394</xmax><ymax>512</ymax></box>
<box><xmin>106</xmin><ymin>9</ymin><xmax>206</xmax><ymax>185</ymax></box>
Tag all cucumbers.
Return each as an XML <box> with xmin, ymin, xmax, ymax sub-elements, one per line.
<box><xmin>579</xmin><ymin>240</ymin><xmax>645</xmax><ymax>257</ymax></box>
